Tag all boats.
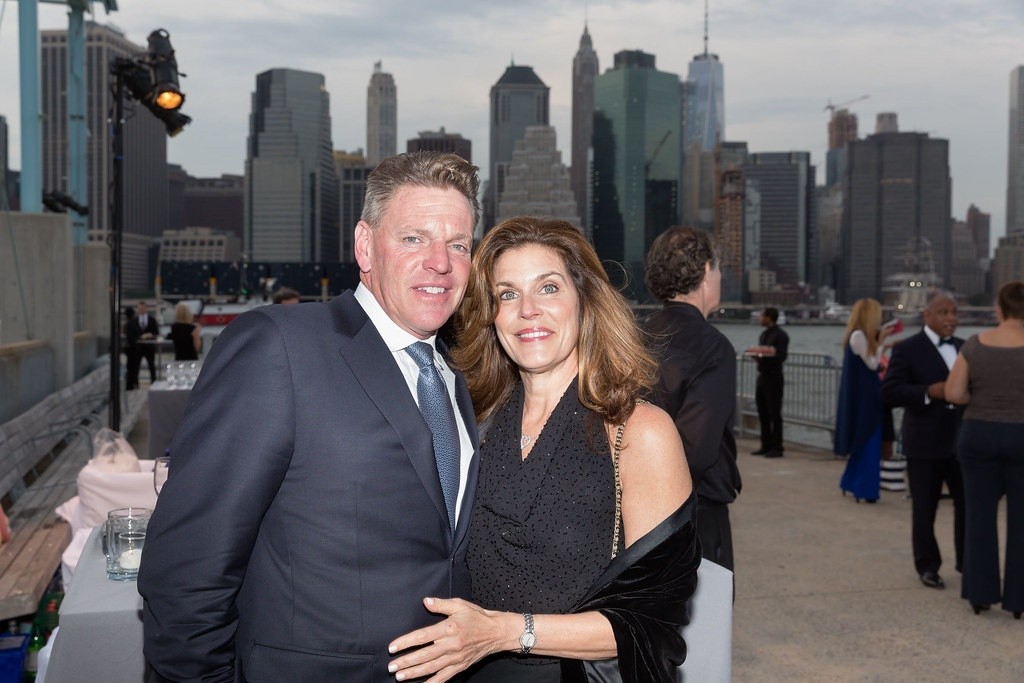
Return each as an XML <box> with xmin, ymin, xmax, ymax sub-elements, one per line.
<box><xmin>752</xmin><ymin>308</ymin><xmax>786</xmax><ymax>326</ymax></box>
<box><xmin>877</xmin><ymin>236</ymin><xmax>945</xmax><ymax>324</ymax></box>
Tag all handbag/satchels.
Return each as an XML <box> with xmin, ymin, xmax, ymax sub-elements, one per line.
<box><xmin>675</xmin><ymin>558</ymin><xmax>733</xmax><ymax>683</ymax></box>
<box><xmin>879</xmin><ymin>457</ymin><xmax>909</xmax><ymax>492</ymax></box>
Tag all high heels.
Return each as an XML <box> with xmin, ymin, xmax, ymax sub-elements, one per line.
<box><xmin>1011</xmin><ymin>610</ymin><xmax>1022</xmax><ymax>619</ymax></box>
<box><xmin>972</xmin><ymin>603</ymin><xmax>989</xmax><ymax>614</ymax></box>
<box><xmin>855</xmin><ymin>495</ymin><xmax>876</xmax><ymax>503</ymax></box>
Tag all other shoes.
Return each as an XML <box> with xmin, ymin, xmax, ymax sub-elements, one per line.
<box><xmin>751</xmin><ymin>444</ymin><xmax>771</xmax><ymax>454</ymax></box>
<box><xmin>765</xmin><ymin>448</ymin><xmax>783</xmax><ymax>457</ymax></box>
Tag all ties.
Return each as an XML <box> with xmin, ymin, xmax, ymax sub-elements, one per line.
<box><xmin>405</xmin><ymin>342</ymin><xmax>460</xmax><ymax>545</ymax></box>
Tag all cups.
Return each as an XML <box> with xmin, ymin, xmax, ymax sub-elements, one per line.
<box><xmin>154</xmin><ymin>456</ymin><xmax>170</xmax><ymax>497</ymax></box>
<box><xmin>166</xmin><ymin>359</ymin><xmax>202</xmax><ymax>389</ymax></box>
<box><xmin>102</xmin><ymin>506</ymin><xmax>153</xmax><ymax>582</ymax></box>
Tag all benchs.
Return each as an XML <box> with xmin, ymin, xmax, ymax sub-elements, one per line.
<box><xmin>0</xmin><ymin>361</ymin><xmax>147</xmax><ymax>635</ymax></box>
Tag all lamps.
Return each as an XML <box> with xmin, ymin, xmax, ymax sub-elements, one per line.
<box><xmin>147</xmin><ymin>28</ymin><xmax>188</xmax><ymax>113</ymax></box>
<box><xmin>107</xmin><ymin>56</ymin><xmax>192</xmax><ymax>137</ymax></box>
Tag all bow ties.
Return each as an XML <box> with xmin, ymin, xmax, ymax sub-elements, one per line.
<box><xmin>939</xmin><ymin>337</ymin><xmax>953</xmax><ymax>346</ymax></box>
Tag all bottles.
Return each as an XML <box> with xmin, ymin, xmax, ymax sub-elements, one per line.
<box><xmin>7</xmin><ymin>591</ymin><xmax>59</xmax><ymax>679</ymax></box>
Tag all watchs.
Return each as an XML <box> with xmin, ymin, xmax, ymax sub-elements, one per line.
<box><xmin>519</xmin><ymin>612</ymin><xmax>537</xmax><ymax>654</ymax></box>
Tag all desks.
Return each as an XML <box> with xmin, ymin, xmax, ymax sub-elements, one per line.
<box><xmin>137</xmin><ymin>339</ymin><xmax>173</xmax><ymax>380</ymax></box>
<box><xmin>42</xmin><ymin>525</ymin><xmax>143</xmax><ymax>683</ymax></box>
<box><xmin>146</xmin><ymin>379</ymin><xmax>193</xmax><ymax>460</ymax></box>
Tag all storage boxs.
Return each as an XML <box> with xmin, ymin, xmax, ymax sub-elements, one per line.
<box><xmin>0</xmin><ymin>632</ymin><xmax>31</xmax><ymax>683</ymax></box>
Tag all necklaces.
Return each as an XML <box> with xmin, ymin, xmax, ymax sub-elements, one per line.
<box><xmin>519</xmin><ymin>427</ymin><xmax>535</xmax><ymax>449</ymax></box>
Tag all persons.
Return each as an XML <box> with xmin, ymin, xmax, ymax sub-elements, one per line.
<box><xmin>633</xmin><ymin>225</ymin><xmax>744</xmax><ymax>606</ymax></box>
<box><xmin>833</xmin><ymin>280</ymin><xmax>1024</xmax><ymax>619</ymax></box>
<box><xmin>388</xmin><ymin>214</ymin><xmax>704</xmax><ymax>683</ymax></box>
<box><xmin>123</xmin><ymin>276</ymin><xmax>299</xmax><ymax>391</ymax></box>
<box><xmin>750</xmin><ymin>308</ymin><xmax>790</xmax><ymax>457</ymax></box>
<box><xmin>136</xmin><ymin>150</ymin><xmax>482</xmax><ymax>683</ymax></box>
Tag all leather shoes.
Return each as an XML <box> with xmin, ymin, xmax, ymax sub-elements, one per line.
<box><xmin>921</xmin><ymin>572</ymin><xmax>945</xmax><ymax>588</ymax></box>
